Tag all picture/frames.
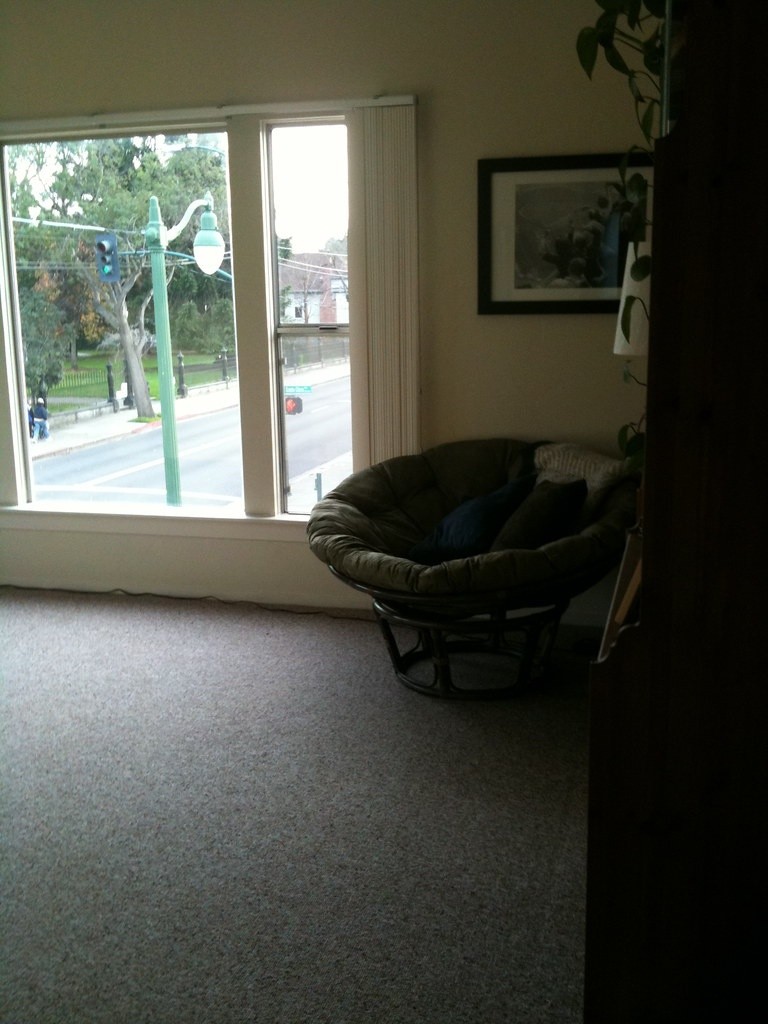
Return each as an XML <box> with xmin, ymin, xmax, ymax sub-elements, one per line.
<box><xmin>476</xmin><ymin>152</ymin><xmax>658</xmax><ymax>316</ymax></box>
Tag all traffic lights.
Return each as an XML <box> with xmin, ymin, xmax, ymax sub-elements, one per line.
<box><xmin>286</xmin><ymin>397</ymin><xmax>302</xmax><ymax>415</ymax></box>
<box><xmin>94</xmin><ymin>232</ymin><xmax>119</xmax><ymax>282</ymax></box>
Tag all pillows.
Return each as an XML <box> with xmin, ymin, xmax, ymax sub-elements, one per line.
<box><xmin>408</xmin><ymin>474</ymin><xmax>539</xmax><ymax>565</ymax></box>
<box><xmin>488</xmin><ymin>478</ymin><xmax>586</xmax><ymax>553</ymax></box>
<box><xmin>535</xmin><ymin>441</ymin><xmax>631</xmax><ymax>511</ymax></box>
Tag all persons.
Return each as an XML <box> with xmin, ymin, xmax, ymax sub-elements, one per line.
<box><xmin>27</xmin><ymin>397</ymin><xmax>50</xmax><ymax>444</ymax></box>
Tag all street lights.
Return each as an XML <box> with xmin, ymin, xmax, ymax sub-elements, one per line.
<box><xmin>143</xmin><ymin>190</ymin><xmax>225</xmax><ymax>505</ymax></box>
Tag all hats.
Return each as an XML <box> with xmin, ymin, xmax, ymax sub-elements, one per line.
<box><xmin>37</xmin><ymin>397</ymin><xmax>45</xmax><ymax>404</ymax></box>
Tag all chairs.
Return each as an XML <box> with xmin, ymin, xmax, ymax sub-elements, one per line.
<box><xmin>307</xmin><ymin>438</ymin><xmax>637</xmax><ymax>701</ymax></box>
